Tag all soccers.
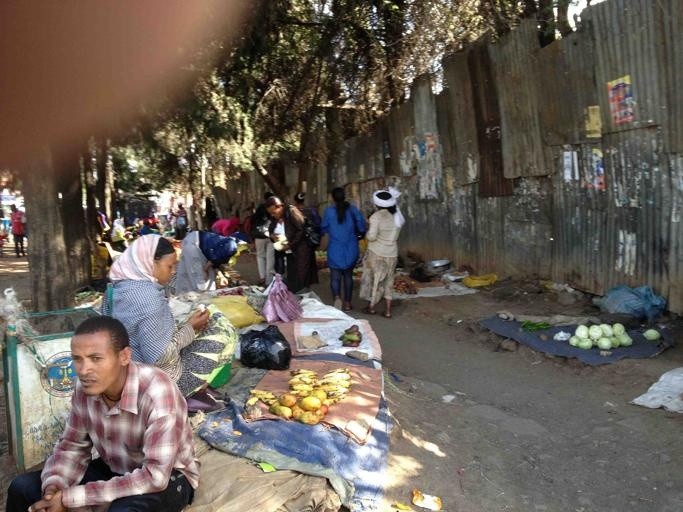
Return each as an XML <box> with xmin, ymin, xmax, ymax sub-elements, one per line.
<box><xmin>394</xmin><ymin>280</ymin><xmax>417</xmax><ymax>295</ymax></box>
<box><xmin>269</xmin><ymin>389</ymin><xmax>326</xmax><ymax>425</ymax></box>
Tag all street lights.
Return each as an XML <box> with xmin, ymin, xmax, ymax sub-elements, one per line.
<box><xmin>177</xmin><ymin>216</ymin><xmax>185</xmax><ymax>229</ymax></box>
<box><xmin>21</xmin><ymin>216</ymin><xmax>27</xmax><ymax>222</ymax></box>
<box><xmin>242</xmin><ymin>272</ymin><xmax>303</xmax><ymax>324</ymax></box>
<box><xmin>303</xmin><ymin>216</ymin><xmax>321</xmax><ymax>251</ymax></box>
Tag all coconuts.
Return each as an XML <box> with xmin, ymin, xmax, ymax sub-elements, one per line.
<box><xmin>338</xmin><ymin>325</ymin><xmax>361</xmax><ymax>347</ymax></box>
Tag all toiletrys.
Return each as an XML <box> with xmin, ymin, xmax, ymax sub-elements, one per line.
<box><xmin>522</xmin><ymin>318</ymin><xmax>553</xmax><ymax>332</ymax></box>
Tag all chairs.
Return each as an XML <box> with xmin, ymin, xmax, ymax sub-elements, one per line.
<box><xmin>333</xmin><ymin>298</ymin><xmax>390</xmax><ymax>318</ymax></box>
<box><xmin>187</xmin><ymin>387</ymin><xmax>225</xmax><ymax>413</ymax></box>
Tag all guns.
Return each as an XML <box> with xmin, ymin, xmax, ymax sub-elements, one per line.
<box><xmin>245</xmin><ymin>388</ymin><xmax>278</xmax><ymax>405</ymax></box>
<box><xmin>289</xmin><ymin>366</ymin><xmax>352</xmax><ymax>404</ymax></box>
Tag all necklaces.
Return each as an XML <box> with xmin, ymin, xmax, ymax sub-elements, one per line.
<box><xmin>104</xmin><ymin>393</ymin><xmax>120</xmax><ymax>401</ymax></box>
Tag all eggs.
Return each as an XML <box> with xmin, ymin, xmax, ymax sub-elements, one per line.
<box><xmin>570</xmin><ymin>323</ymin><xmax>633</xmax><ymax>350</ymax></box>
<box><xmin>643</xmin><ymin>329</ymin><xmax>661</xmax><ymax>341</ymax></box>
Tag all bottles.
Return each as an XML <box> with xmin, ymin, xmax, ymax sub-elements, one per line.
<box><xmin>262</xmin><ymin>195</ymin><xmax>284</xmax><ymax>207</ymax></box>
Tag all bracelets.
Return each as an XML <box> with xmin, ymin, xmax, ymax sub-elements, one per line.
<box><xmin>431</xmin><ymin>258</ymin><xmax>451</xmax><ymax>272</ymax></box>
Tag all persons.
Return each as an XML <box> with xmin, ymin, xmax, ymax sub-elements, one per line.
<box><xmin>359</xmin><ymin>190</ymin><xmax>404</xmax><ymax>318</ymax></box>
<box><xmin>170</xmin><ymin>204</ymin><xmax>189</xmax><ymax>240</ymax></box>
<box><xmin>9</xmin><ymin>204</ymin><xmax>27</xmax><ymax>258</ymax></box>
<box><xmin>198</xmin><ymin>230</ymin><xmax>237</xmax><ymax>278</ymax></box>
<box><xmin>96</xmin><ymin>211</ymin><xmax>112</xmax><ymax>242</ymax></box>
<box><xmin>294</xmin><ymin>190</ymin><xmax>323</xmax><ymax>289</ymax></box>
<box><xmin>265</xmin><ymin>197</ymin><xmax>305</xmax><ymax>294</ymax></box>
<box><xmin>177</xmin><ymin>231</ymin><xmax>217</xmax><ymax>292</ymax></box>
<box><xmin>123</xmin><ymin>206</ymin><xmax>160</xmax><ymax>236</ymax></box>
<box><xmin>5</xmin><ymin>315</ymin><xmax>203</xmax><ymax>511</ymax></box>
<box><xmin>112</xmin><ymin>219</ymin><xmax>132</xmax><ymax>251</ymax></box>
<box><xmin>321</xmin><ymin>187</ymin><xmax>366</xmax><ymax>311</ymax></box>
<box><xmin>17</xmin><ymin>200</ymin><xmax>27</xmax><ymax>237</ymax></box>
<box><xmin>102</xmin><ymin>234</ymin><xmax>211</xmax><ymax>383</ymax></box>
<box><xmin>204</xmin><ymin>197</ymin><xmax>255</xmax><ymax>243</ymax></box>
<box><xmin>253</xmin><ymin>191</ymin><xmax>278</xmax><ymax>286</ymax></box>
